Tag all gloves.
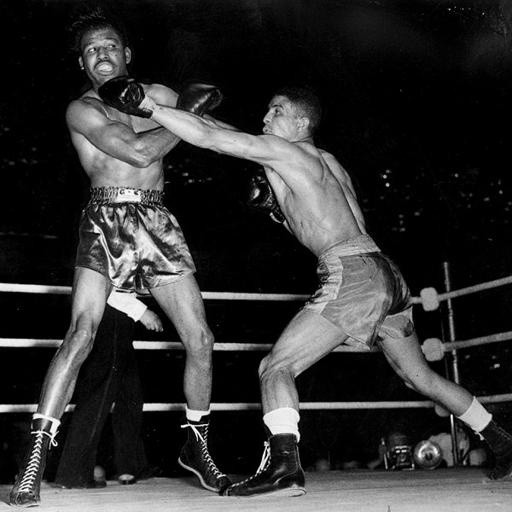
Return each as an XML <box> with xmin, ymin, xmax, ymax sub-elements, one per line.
<box><xmin>97</xmin><ymin>74</ymin><xmax>156</xmax><ymax>123</ymax></box>
<box><xmin>232</xmin><ymin>163</ymin><xmax>287</xmax><ymax>225</ymax></box>
<box><xmin>175</xmin><ymin>81</ymin><xmax>225</xmax><ymax>120</ymax></box>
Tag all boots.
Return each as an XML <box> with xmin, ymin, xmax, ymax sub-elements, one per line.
<box><xmin>222</xmin><ymin>423</ymin><xmax>307</xmax><ymax>498</ymax></box>
<box><xmin>479</xmin><ymin>416</ymin><xmax>512</xmax><ymax>482</ymax></box>
<box><xmin>8</xmin><ymin>409</ymin><xmax>62</xmax><ymax>509</ymax></box>
<box><xmin>170</xmin><ymin>413</ymin><xmax>234</xmax><ymax>494</ymax></box>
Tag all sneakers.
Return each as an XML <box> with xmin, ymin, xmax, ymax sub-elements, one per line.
<box><xmin>127</xmin><ymin>467</ymin><xmax>160</xmax><ymax>481</ymax></box>
<box><xmin>49</xmin><ymin>476</ymin><xmax>107</xmax><ymax>489</ymax></box>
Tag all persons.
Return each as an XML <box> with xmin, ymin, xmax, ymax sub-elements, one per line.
<box><xmin>53</xmin><ymin>288</ymin><xmax>165</xmax><ymax>492</ymax></box>
<box><xmin>341</xmin><ymin>457</ymin><xmax>360</xmax><ymax>470</ymax></box>
<box><xmin>93</xmin><ymin>465</ymin><xmax>106</xmax><ymax>481</ymax></box>
<box><xmin>315</xmin><ymin>456</ymin><xmax>330</xmax><ymax>470</ymax></box>
<box><xmin>95</xmin><ymin>74</ymin><xmax>512</xmax><ymax>497</ymax></box>
<box><xmin>8</xmin><ymin>4</ymin><xmax>232</xmax><ymax>509</ymax></box>
<box><xmin>366</xmin><ymin>444</ymin><xmax>387</xmax><ymax>468</ymax></box>
